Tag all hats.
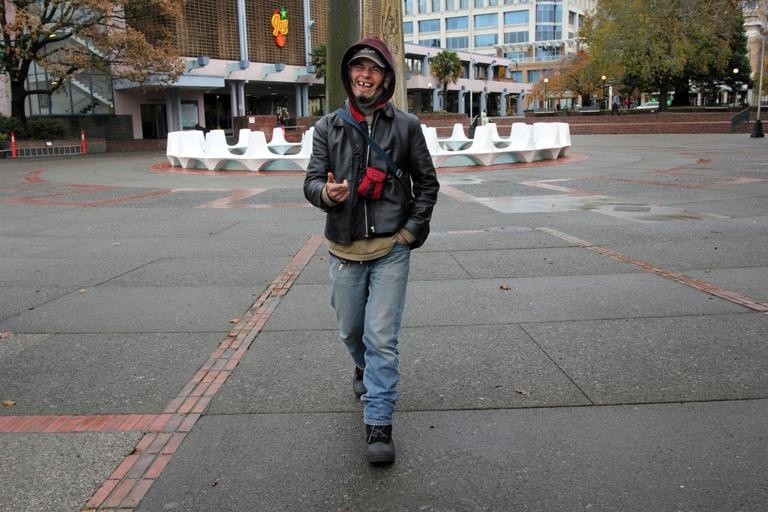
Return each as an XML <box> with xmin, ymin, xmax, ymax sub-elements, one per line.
<box><xmin>347</xmin><ymin>47</ymin><xmax>385</xmax><ymax>68</ymax></box>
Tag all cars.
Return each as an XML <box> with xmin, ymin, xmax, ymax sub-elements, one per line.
<box><xmin>629</xmin><ymin>101</ymin><xmax>660</xmax><ymax>109</ymax></box>
<box><xmin>728</xmin><ymin>98</ymin><xmax>747</xmax><ymax>108</ymax></box>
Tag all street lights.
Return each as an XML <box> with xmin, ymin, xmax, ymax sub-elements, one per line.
<box><xmin>428</xmin><ymin>84</ymin><xmax>433</xmax><ymax>114</ymax></box>
<box><xmin>601</xmin><ymin>75</ymin><xmax>606</xmax><ymax>111</ymax></box>
<box><xmin>544</xmin><ymin>78</ymin><xmax>548</xmax><ymax>111</ymax></box>
<box><xmin>746</xmin><ymin>30</ymin><xmax>765</xmax><ymax>138</ymax></box>
<box><xmin>732</xmin><ymin>68</ymin><xmax>740</xmax><ymax>109</ymax></box>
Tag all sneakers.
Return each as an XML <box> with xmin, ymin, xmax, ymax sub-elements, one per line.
<box><xmin>351</xmin><ymin>365</ymin><xmax>368</xmax><ymax>397</ymax></box>
<box><xmin>366</xmin><ymin>425</ymin><xmax>395</xmax><ymax>463</ymax></box>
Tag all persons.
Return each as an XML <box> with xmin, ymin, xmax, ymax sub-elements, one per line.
<box><xmin>480</xmin><ymin>108</ymin><xmax>489</xmax><ymax>126</ymax></box>
<box><xmin>610</xmin><ymin>91</ymin><xmax>641</xmax><ymax>116</ymax></box>
<box><xmin>274</xmin><ymin>108</ymin><xmax>291</xmax><ymax>125</ymax></box>
<box><xmin>556</xmin><ymin>102</ymin><xmax>561</xmax><ymax>111</ymax></box>
<box><xmin>304</xmin><ymin>38</ymin><xmax>439</xmax><ymax>465</ymax></box>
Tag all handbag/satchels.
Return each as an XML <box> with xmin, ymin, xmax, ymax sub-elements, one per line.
<box><xmin>358</xmin><ymin>167</ymin><xmax>386</xmax><ymax>201</ymax></box>
